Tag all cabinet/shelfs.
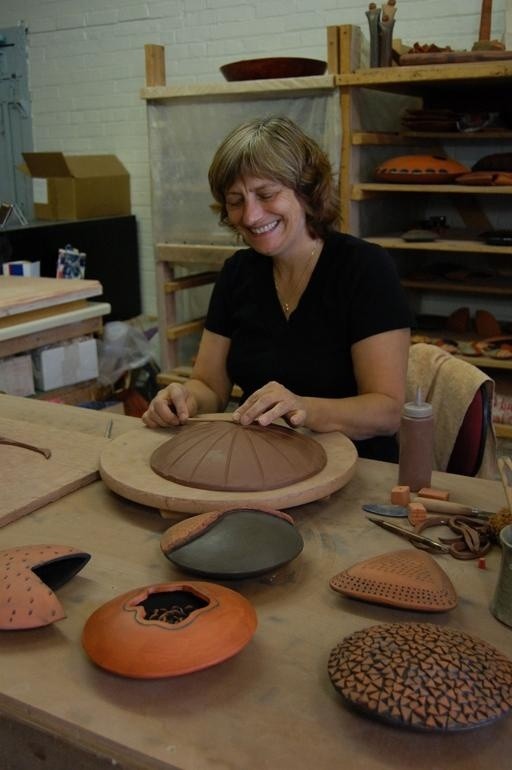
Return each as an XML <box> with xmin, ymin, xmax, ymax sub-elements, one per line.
<box><xmin>135</xmin><ymin>25</ymin><xmax>341</xmax><ymax>401</ymax></box>
<box><xmin>341</xmin><ymin>20</ymin><xmax>511</xmax><ymax>442</ymax></box>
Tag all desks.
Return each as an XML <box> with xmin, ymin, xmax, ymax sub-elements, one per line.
<box><xmin>1</xmin><ymin>389</ymin><xmax>511</xmax><ymax>768</ymax></box>
<box><xmin>3</xmin><ymin>214</ymin><xmax>143</xmax><ymax>329</ymax></box>
<box><xmin>1</xmin><ymin>258</ymin><xmax>115</xmax><ymax>407</ymax></box>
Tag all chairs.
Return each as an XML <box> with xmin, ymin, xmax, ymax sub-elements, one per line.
<box><xmin>376</xmin><ymin>339</ymin><xmax>496</xmax><ymax>481</ymax></box>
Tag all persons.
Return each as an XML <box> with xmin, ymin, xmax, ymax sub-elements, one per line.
<box><xmin>142</xmin><ymin>113</ymin><xmax>410</xmax><ymax>440</ymax></box>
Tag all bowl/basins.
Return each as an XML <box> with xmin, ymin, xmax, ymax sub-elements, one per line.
<box><xmin>219</xmin><ymin>56</ymin><xmax>328</xmax><ymax>85</ymax></box>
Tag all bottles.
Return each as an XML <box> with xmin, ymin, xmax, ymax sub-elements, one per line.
<box><xmin>399</xmin><ymin>382</ymin><xmax>437</xmax><ymax>490</ymax></box>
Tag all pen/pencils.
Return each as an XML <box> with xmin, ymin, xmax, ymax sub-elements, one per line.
<box><xmin>370</xmin><ymin>518</ymin><xmax>445</xmax><ymax>550</ymax></box>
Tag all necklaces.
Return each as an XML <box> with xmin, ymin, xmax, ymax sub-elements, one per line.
<box><xmin>267</xmin><ymin>246</ymin><xmax>319</xmax><ymax>312</ymax></box>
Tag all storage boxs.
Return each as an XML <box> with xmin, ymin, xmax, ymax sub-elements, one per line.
<box><xmin>15</xmin><ymin>146</ymin><xmax>136</xmax><ymax>222</ymax></box>
